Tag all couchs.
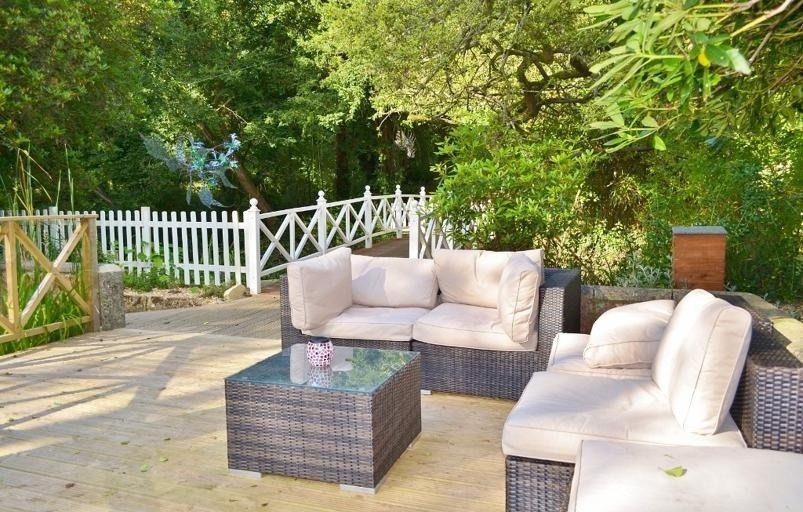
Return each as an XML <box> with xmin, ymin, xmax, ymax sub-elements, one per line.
<box><xmin>502</xmin><ymin>294</ymin><xmax>802</xmax><ymax>512</ymax></box>
<box><xmin>280</xmin><ymin>267</ymin><xmax>581</xmax><ymax>399</ymax></box>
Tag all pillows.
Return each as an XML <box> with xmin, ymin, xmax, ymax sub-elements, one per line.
<box><xmin>583</xmin><ymin>300</ymin><xmax>676</xmax><ymax>369</ymax></box>
<box><xmin>500</xmin><ymin>253</ymin><xmax>538</xmax><ymax>343</ymax></box>
<box><xmin>429</xmin><ymin>248</ymin><xmax>545</xmax><ymax>308</ymax></box>
<box><xmin>350</xmin><ymin>255</ymin><xmax>440</xmax><ymax>309</ymax></box>
<box><xmin>286</xmin><ymin>247</ymin><xmax>352</xmax><ymax>330</ymax></box>
<box><xmin>648</xmin><ymin>288</ymin><xmax>714</xmax><ymax>399</ymax></box>
<box><xmin>668</xmin><ymin>298</ymin><xmax>754</xmax><ymax>435</ymax></box>
